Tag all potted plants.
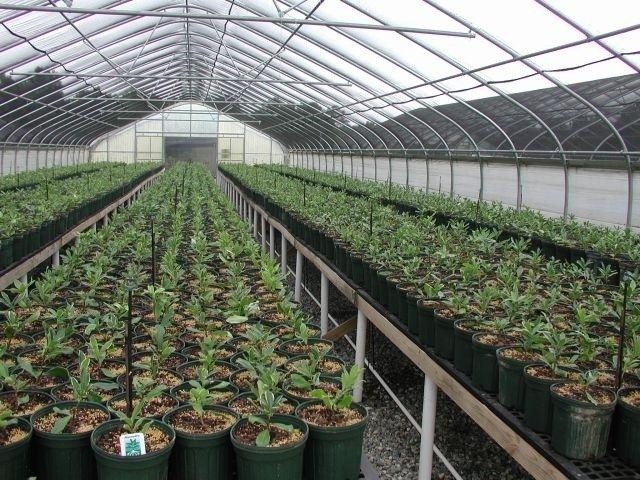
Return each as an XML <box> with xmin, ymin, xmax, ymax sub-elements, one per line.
<box><xmin>1</xmin><ymin>158</ymin><xmax>640</xmax><ymax>478</ymax></box>
